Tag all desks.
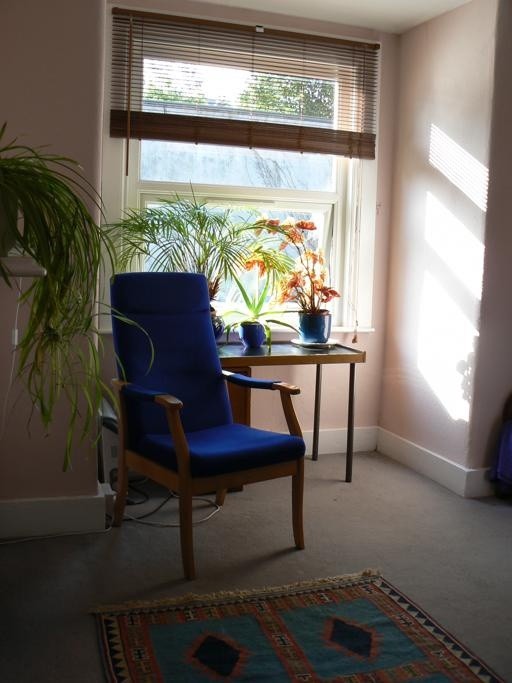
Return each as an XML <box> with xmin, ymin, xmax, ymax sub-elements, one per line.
<box><xmin>213</xmin><ymin>338</ymin><xmax>364</xmax><ymax>482</ymax></box>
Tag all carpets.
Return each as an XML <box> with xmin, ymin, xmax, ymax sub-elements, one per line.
<box><xmin>90</xmin><ymin>568</ymin><xmax>509</xmax><ymax>683</ymax></box>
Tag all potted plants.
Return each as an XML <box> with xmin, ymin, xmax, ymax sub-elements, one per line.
<box><xmin>218</xmin><ymin>248</ymin><xmax>302</xmax><ymax>353</ymax></box>
<box><xmin>245</xmin><ymin>218</ymin><xmax>340</xmax><ymax>342</ymax></box>
<box><xmin>102</xmin><ymin>179</ymin><xmax>296</xmax><ymax>342</ymax></box>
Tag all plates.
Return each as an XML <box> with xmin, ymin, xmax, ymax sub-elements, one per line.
<box><xmin>290</xmin><ymin>338</ymin><xmax>338</xmax><ymax>351</ymax></box>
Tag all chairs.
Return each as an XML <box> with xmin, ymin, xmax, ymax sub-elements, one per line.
<box><xmin>109</xmin><ymin>273</ymin><xmax>305</xmax><ymax>580</ymax></box>
